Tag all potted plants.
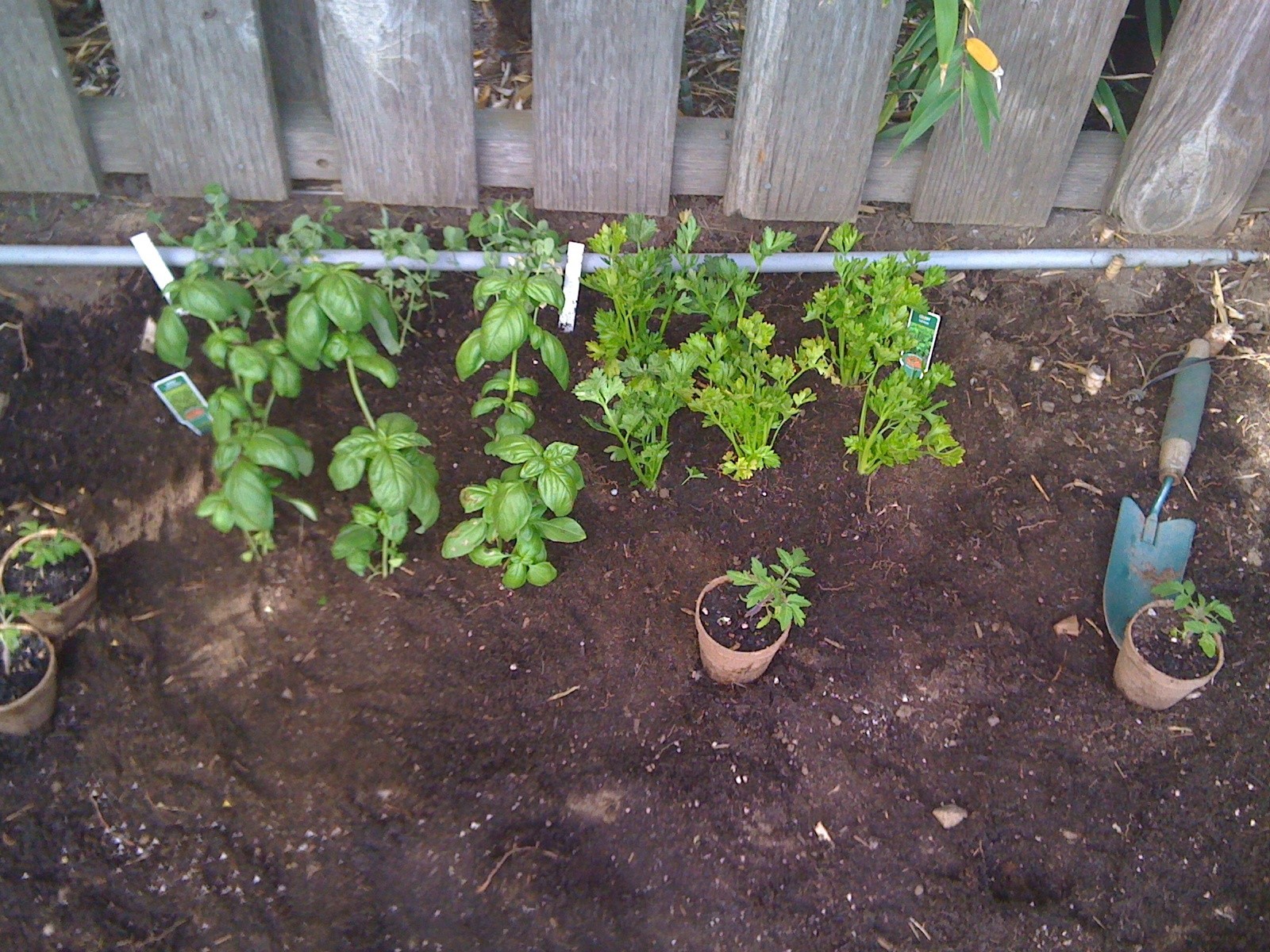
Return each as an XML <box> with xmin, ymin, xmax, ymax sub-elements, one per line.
<box><xmin>1114</xmin><ymin>578</ymin><xmax>1236</xmax><ymax>711</ymax></box>
<box><xmin>0</xmin><ymin>521</ymin><xmax>98</xmax><ymax>739</ymax></box>
<box><xmin>693</xmin><ymin>544</ymin><xmax>816</xmax><ymax>684</ymax></box>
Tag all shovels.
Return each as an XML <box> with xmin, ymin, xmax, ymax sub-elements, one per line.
<box><xmin>1102</xmin><ymin>339</ymin><xmax>1210</xmax><ymax>650</ymax></box>
